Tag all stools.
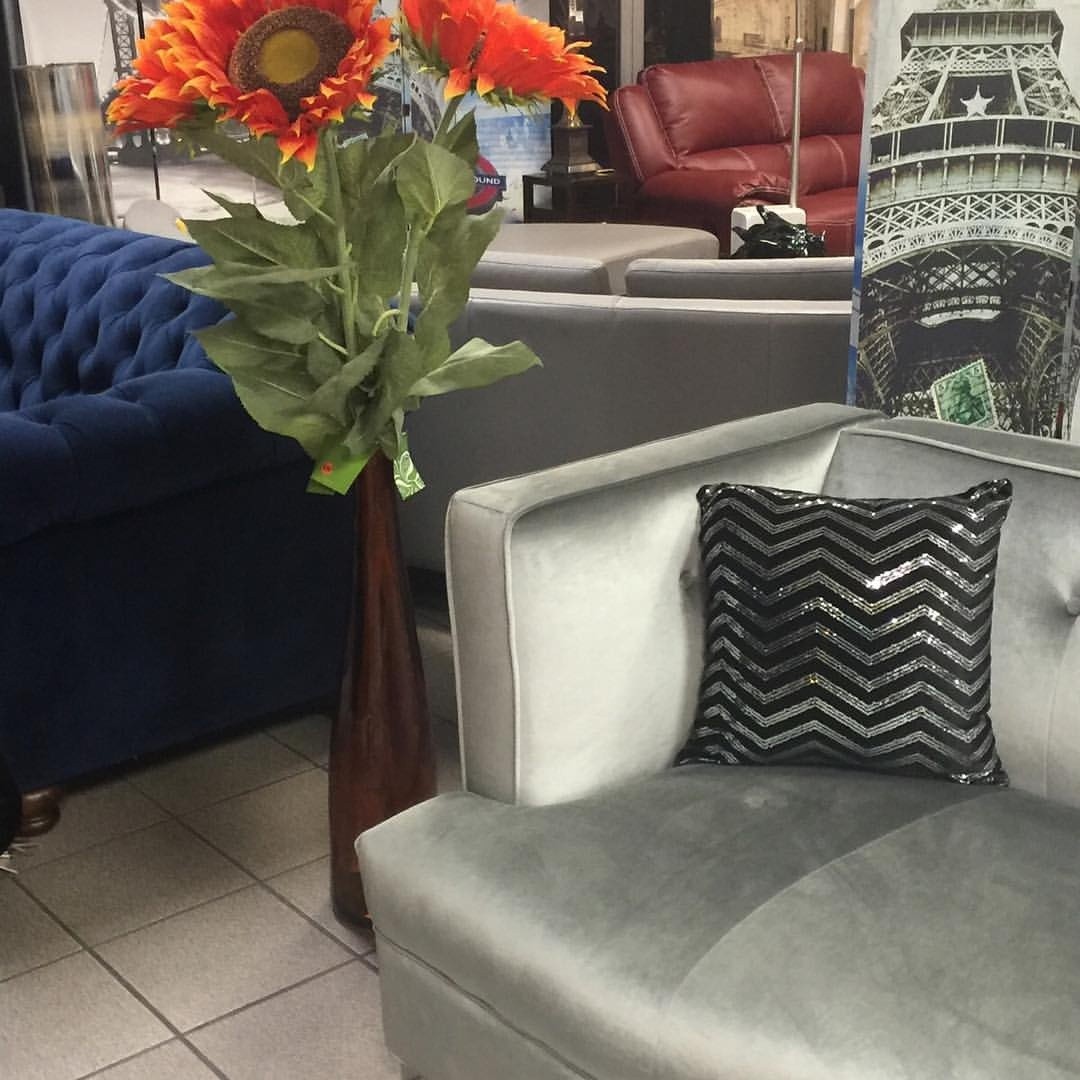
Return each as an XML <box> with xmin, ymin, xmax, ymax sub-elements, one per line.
<box><xmin>485</xmin><ymin>222</ymin><xmax>718</xmax><ymax>298</ymax></box>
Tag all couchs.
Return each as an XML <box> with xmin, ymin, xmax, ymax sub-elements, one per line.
<box><xmin>0</xmin><ymin>52</ymin><xmax>1080</xmax><ymax>1080</ymax></box>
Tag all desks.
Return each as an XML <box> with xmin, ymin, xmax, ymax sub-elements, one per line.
<box><xmin>522</xmin><ymin>172</ymin><xmax>621</xmax><ymax>223</ymax></box>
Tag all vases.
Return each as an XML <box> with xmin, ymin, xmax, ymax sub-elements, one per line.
<box><xmin>331</xmin><ymin>455</ymin><xmax>435</xmax><ymax>931</ymax></box>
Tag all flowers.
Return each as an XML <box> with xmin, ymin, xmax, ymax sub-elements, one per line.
<box><xmin>105</xmin><ymin>0</ymin><xmax>608</xmax><ymax>494</ymax></box>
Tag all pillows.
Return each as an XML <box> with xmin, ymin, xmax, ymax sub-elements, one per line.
<box><xmin>674</xmin><ymin>480</ymin><xmax>1015</xmax><ymax>784</ymax></box>
